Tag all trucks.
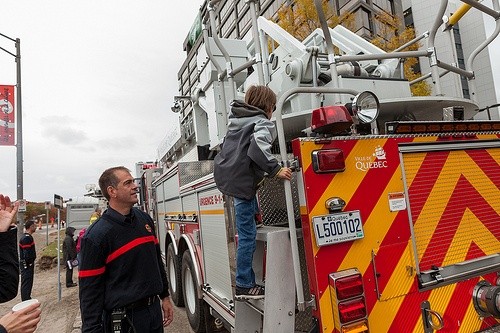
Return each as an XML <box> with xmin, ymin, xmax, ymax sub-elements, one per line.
<box><xmin>83</xmin><ymin>0</ymin><xmax>500</xmax><ymax>333</ymax></box>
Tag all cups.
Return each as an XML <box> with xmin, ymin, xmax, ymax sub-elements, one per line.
<box><xmin>12</xmin><ymin>299</ymin><xmax>40</xmax><ymax>329</ymax></box>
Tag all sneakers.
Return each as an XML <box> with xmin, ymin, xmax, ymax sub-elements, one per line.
<box><xmin>235</xmin><ymin>284</ymin><xmax>265</xmax><ymax>300</ymax></box>
<box><xmin>255</xmin><ymin>216</ymin><xmax>263</xmax><ymax>229</ymax></box>
<box><xmin>66</xmin><ymin>283</ymin><xmax>77</xmax><ymax>288</ymax></box>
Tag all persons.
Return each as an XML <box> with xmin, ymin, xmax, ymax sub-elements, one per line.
<box><xmin>19</xmin><ymin>221</ymin><xmax>37</xmax><ymax>302</ymax></box>
<box><xmin>63</xmin><ymin>227</ymin><xmax>78</xmax><ymax>287</ymax></box>
<box><xmin>78</xmin><ymin>166</ymin><xmax>174</xmax><ymax>333</ymax></box>
<box><xmin>0</xmin><ymin>194</ymin><xmax>42</xmax><ymax>333</ymax></box>
<box><xmin>214</xmin><ymin>85</ymin><xmax>292</xmax><ymax>302</ymax></box>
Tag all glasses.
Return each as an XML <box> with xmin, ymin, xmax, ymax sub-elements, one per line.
<box><xmin>271</xmin><ymin>105</ymin><xmax>276</xmax><ymax>112</ymax></box>
